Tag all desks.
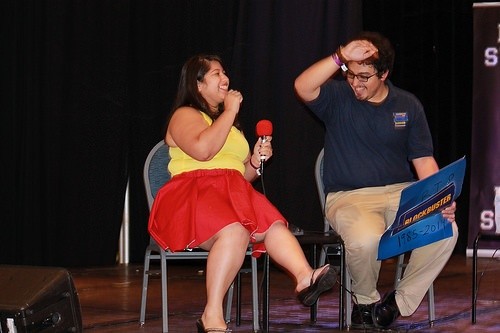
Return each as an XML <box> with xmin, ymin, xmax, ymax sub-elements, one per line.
<box><xmin>263</xmin><ymin>231</ymin><xmax>347</xmax><ymax>332</ymax></box>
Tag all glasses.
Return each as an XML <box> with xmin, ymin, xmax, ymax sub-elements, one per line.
<box><xmin>342</xmin><ymin>67</ymin><xmax>384</xmax><ymax>83</ymax></box>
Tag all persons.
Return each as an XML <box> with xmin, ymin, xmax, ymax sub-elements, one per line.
<box><xmin>147</xmin><ymin>53</ymin><xmax>337</xmax><ymax>333</ymax></box>
<box><xmin>294</xmin><ymin>30</ymin><xmax>460</xmax><ymax>330</ymax></box>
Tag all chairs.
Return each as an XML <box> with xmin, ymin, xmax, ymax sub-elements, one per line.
<box><xmin>140</xmin><ymin>137</ymin><xmax>270</xmax><ymax>333</ymax></box>
<box><xmin>315</xmin><ymin>141</ymin><xmax>435</xmax><ymax>332</ymax></box>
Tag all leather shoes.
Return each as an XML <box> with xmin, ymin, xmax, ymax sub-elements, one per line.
<box><xmin>351</xmin><ymin>304</ymin><xmax>374</xmax><ymax>329</ymax></box>
<box><xmin>372</xmin><ymin>289</ymin><xmax>401</xmax><ymax>328</ymax></box>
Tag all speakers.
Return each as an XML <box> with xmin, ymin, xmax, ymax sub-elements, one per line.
<box><xmin>0</xmin><ymin>263</ymin><xmax>82</xmax><ymax>333</ymax></box>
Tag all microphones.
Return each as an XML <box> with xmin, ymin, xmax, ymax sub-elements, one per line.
<box><xmin>256</xmin><ymin>120</ymin><xmax>273</xmax><ymax>175</ymax></box>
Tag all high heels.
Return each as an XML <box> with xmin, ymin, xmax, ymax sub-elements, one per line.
<box><xmin>296</xmin><ymin>263</ymin><xmax>339</xmax><ymax>307</ymax></box>
<box><xmin>195</xmin><ymin>319</ymin><xmax>233</xmax><ymax>333</ymax></box>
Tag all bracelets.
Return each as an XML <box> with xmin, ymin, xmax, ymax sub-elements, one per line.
<box><xmin>333</xmin><ymin>45</ymin><xmax>348</xmax><ymax>68</ymax></box>
<box><xmin>250</xmin><ymin>158</ymin><xmax>261</xmax><ymax>170</ymax></box>
